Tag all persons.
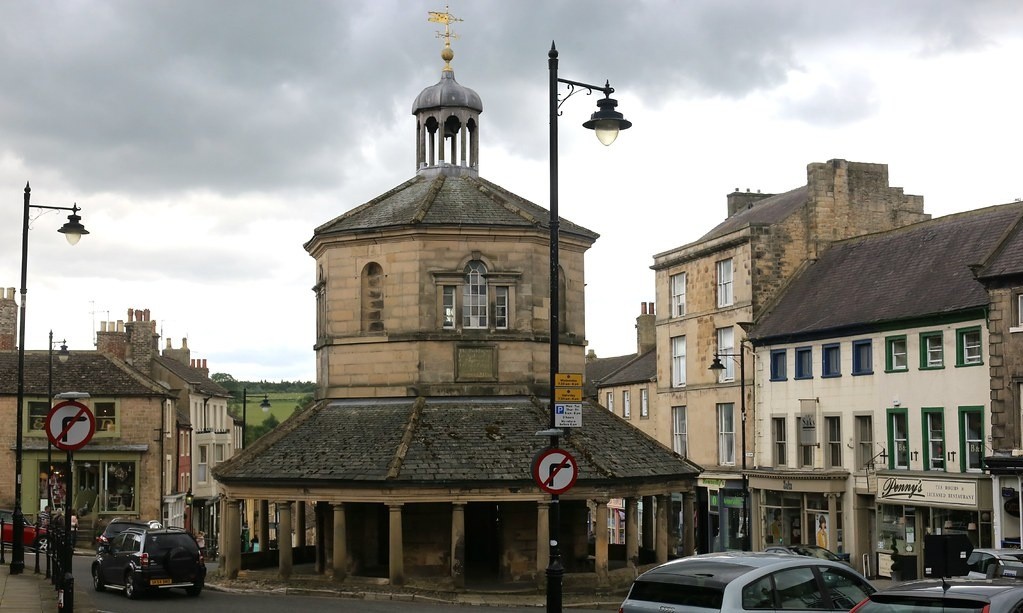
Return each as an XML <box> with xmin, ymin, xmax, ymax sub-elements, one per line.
<box><xmin>38</xmin><ymin>506</ymin><xmax>52</xmax><ymax>528</ymax></box>
<box><xmin>71</xmin><ymin>509</ymin><xmax>80</xmax><ymax>552</ymax></box>
<box><xmin>770</xmin><ymin>508</ymin><xmax>782</xmax><ymax>543</ymax></box>
<box><xmin>817</xmin><ymin>515</ymin><xmax>828</xmax><ymax>549</ymax></box>
<box><xmin>195</xmin><ymin>530</ymin><xmax>206</xmax><ymax>558</ymax></box>
<box><xmin>51</xmin><ymin>508</ymin><xmax>65</xmax><ymax>528</ymax></box>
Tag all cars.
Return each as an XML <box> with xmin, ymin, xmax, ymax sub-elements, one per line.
<box><xmin>847</xmin><ymin>577</ymin><xmax>1023</xmax><ymax>613</ymax></box>
<box><xmin>961</xmin><ymin>548</ymin><xmax>1023</xmax><ymax>581</ymax></box>
<box><xmin>618</xmin><ymin>550</ymin><xmax>895</xmax><ymax>613</ymax></box>
<box><xmin>762</xmin><ymin>544</ymin><xmax>860</xmax><ymax>589</ymax></box>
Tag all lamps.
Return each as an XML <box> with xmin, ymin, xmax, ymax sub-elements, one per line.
<box><xmin>967</xmin><ymin>512</ymin><xmax>976</xmax><ymax>531</ymax></box>
<box><xmin>944</xmin><ymin>510</ymin><xmax>952</xmax><ymax>528</ymax></box>
<box><xmin>184</xmin><ymin>488</ymin><xmax>194</xmax><ymax>506</ymax></box>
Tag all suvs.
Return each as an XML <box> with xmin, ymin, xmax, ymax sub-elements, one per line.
<box><xmin>98</xmin><ymin>518</ymin><xmax>163</xmax><ymax>557</ymax></box>
<box><xmin>91</xmin><ymin>525</ymin><xmax>207</xmax><ymax>600</ymax></box>
<box><xmin>0</xmin><ymin>508</ymin><xmax>54</xmax><ymax>554</ymax></box>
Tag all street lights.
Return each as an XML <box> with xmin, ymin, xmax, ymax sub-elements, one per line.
<box><xmin>708</xmin><ymin>336</ymin><xmax>749</xmax><ymax>551</ymax></box>
<box><xmin>7</xmin><ymin>178</ymin><xmax>90</xmax><ymax>569</ymax></box>
<box><xmin>52</xmin><ymin>392</ymin><xmax>90</xmax><ymax>613</ymax></box>
<box><xmin>535</xmin><ymin>35</ymin><xmax>632</xmax><ymax>613</ymax></box>
